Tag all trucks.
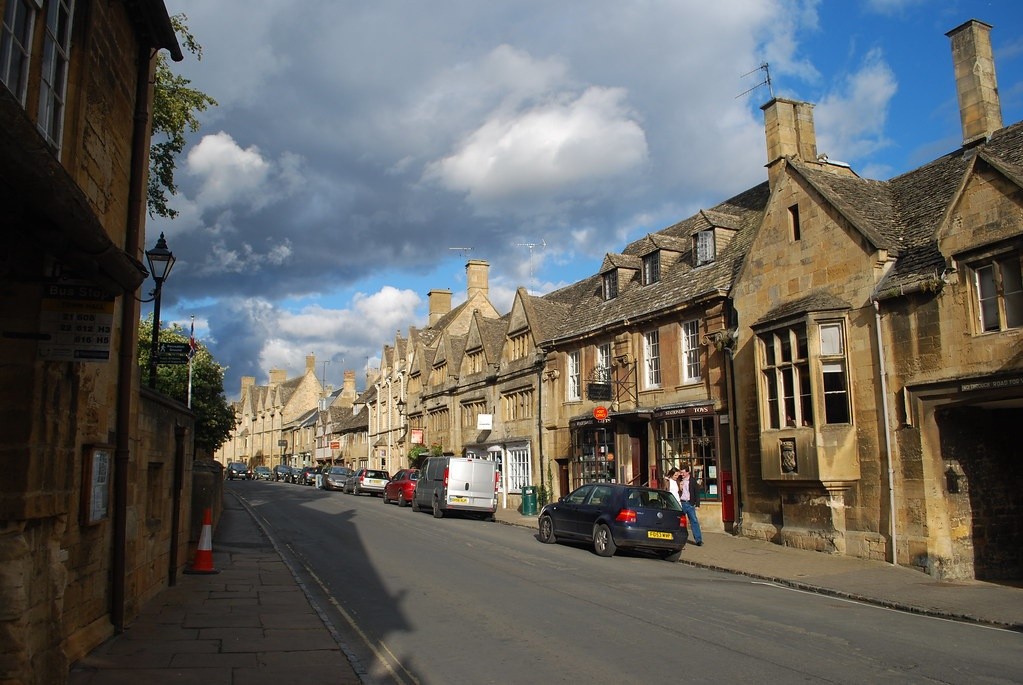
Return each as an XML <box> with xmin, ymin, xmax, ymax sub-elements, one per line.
<box><xmin>411</xmin><ymin>456</ymin><xmax>499</xmax><ymax>522</ymax></box>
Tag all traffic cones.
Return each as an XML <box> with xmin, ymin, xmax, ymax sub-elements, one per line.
<box><xmin>183</xmin><ymin>507</ymin><xmax>222</xmax><ymax>574</ymax></box>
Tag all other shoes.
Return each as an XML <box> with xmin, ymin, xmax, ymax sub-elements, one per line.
<box><xmin>698</xmin><ymin>541</ymin><xmax>704</xmax><ymax>545</ymax></box>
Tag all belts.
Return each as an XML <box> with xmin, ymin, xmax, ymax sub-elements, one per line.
<box><xmin>681</xmin><ymin>500</ymin><xmax>690</xmax><ymax>502</ymax></box>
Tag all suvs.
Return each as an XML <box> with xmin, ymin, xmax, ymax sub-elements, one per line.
<box><xmin>225</xmin><ymin>462</ymin><xmax>249</xmax><ymax>480</ymax></box>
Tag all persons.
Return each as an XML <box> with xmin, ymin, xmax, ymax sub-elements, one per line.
<box><xmin>662</xmin><ymin>465</ymin><xmax>704</xmax><ymax>546</ymax></box>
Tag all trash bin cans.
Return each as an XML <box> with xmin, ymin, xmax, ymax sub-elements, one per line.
<box><xmin>522</xmin><ymin>486</ymin><xmax>537</xmax><ymax>516</ymax></box>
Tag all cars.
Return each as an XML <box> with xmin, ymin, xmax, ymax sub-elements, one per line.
<box><xmin>537</xmin><ymin>481</ymin><xmax>689</xmax><ymax>562</ymax></box>
<box><xmin>343</xmin><ymin>467</ymin><xmax>391</xmax><ymax>497</ymax></box>
<box><xmin>291</xmin><ymin>468</ymin><xmax>302</xmax><ymax>481</ymax></box>
<box><xmin>382</xmin><ymin>469</ymin><xmax>420</xmax><ymax>507</ymax></box>
<box><xmin>322</xmin><ymin>465</ymin><xmax>353</xmax><ymax>491</ymax></box>
<box><xmin>253</xmin><ymin>466</ymin><xmax>272</xmax><ymax>481</ymax></box>
<box><xmin>273</xmin><ymin>464</ymin><xmax>292</xmax><ymax>483</ymax></box>
<box><xmin>297</xmin><ymin>466</ymin><xmax>318</xmax><ymax>486</ymax></box>
